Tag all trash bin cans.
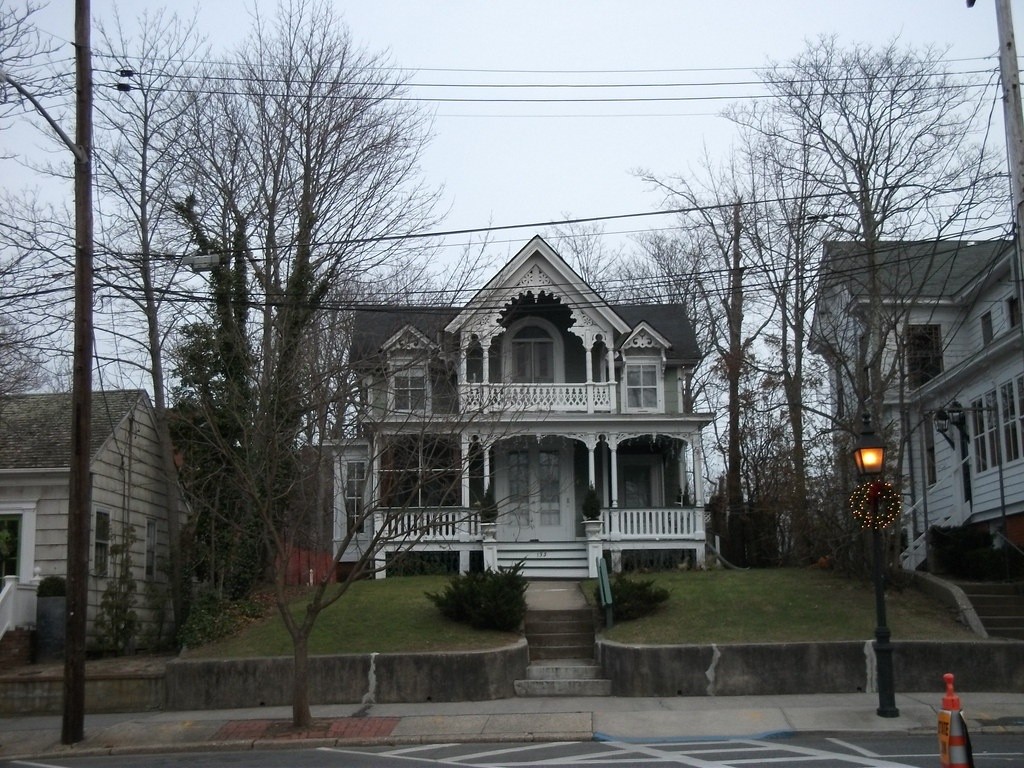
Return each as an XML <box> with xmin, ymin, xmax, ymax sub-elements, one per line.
<box><xmin>35</xmin><ymin>574</ymin><xmax>68</xmax><ymax>666</ymax></box>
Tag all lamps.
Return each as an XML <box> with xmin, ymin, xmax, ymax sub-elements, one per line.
<box><xmin>934</xmin><ymin>408</ymin><xmax>955</xmax><ymax>449</ymax></box>
<box><xmin>949</xmin><ymin>398</ymin><xmax>970</xmax><ymax>443</ymax></box>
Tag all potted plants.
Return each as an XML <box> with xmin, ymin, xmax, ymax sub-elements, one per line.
<box><xmin>476</xmin><ymin>483</ymin><xmax>501</xmax><ymax>543</ymax></box>
<box><xmin>580</xmin><ymin>481</ymin><xmax>605</xmax><ymax>542</ymax></box>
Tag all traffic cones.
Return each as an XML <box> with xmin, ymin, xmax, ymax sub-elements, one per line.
<box><xmin>941</xmin><ymin>672</ymin><xmax>970</xmax><ymax>768</ymax></box>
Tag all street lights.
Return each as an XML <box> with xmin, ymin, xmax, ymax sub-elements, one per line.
<box><xmin>852</xmin><ymin>413</ymin><xmax>900</xmax><ymax>719</ymax></box>
